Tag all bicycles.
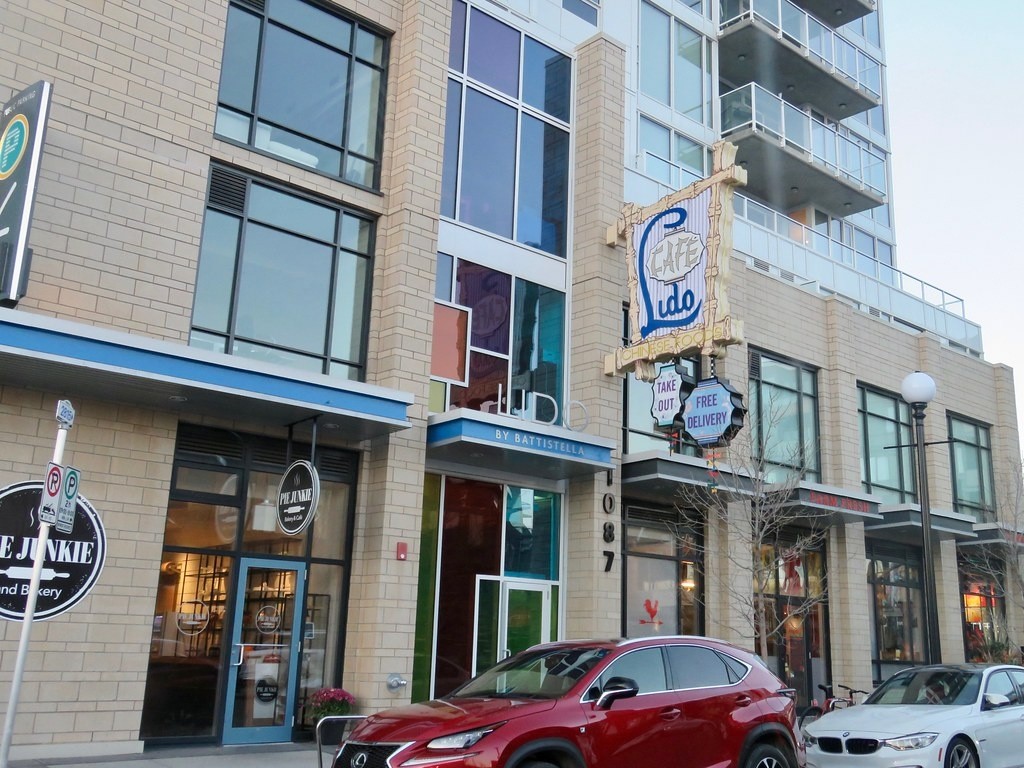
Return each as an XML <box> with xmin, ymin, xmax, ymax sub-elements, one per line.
<box><xmin>798</xmin><ymin>684</ymin><xmax>868</xmax><ymax>747</ymax></box>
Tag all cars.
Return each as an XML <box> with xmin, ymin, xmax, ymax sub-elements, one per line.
<box><xmin>139</xmin><ymin>655</ymin><xmax>246</xmax><ymax>734</ymax></box>
<box><xmin>414</xmin><ymin>651</ymin><xmax>473</xmax><ymax>702</ymax></box>
<box><xmin>802</xmin><ymin>663</ymin><xmax>1024</xmax><ymax>768</ymax></box>
<box><xmin>329</xmin><ymin>638</ymin><xmax>802</xmax><ymax>768</ymax></box>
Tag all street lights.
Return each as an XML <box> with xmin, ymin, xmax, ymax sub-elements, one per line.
<box><xmin>882</xmin><ymin>370</ymin><xmax>957</xmax><ymax>665</ymax></box>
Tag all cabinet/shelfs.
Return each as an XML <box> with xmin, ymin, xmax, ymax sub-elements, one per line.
<box><xmin>191</xmin><ymin>535</ymin><xmax>302</xmax><ymax>659</ymax></box>
